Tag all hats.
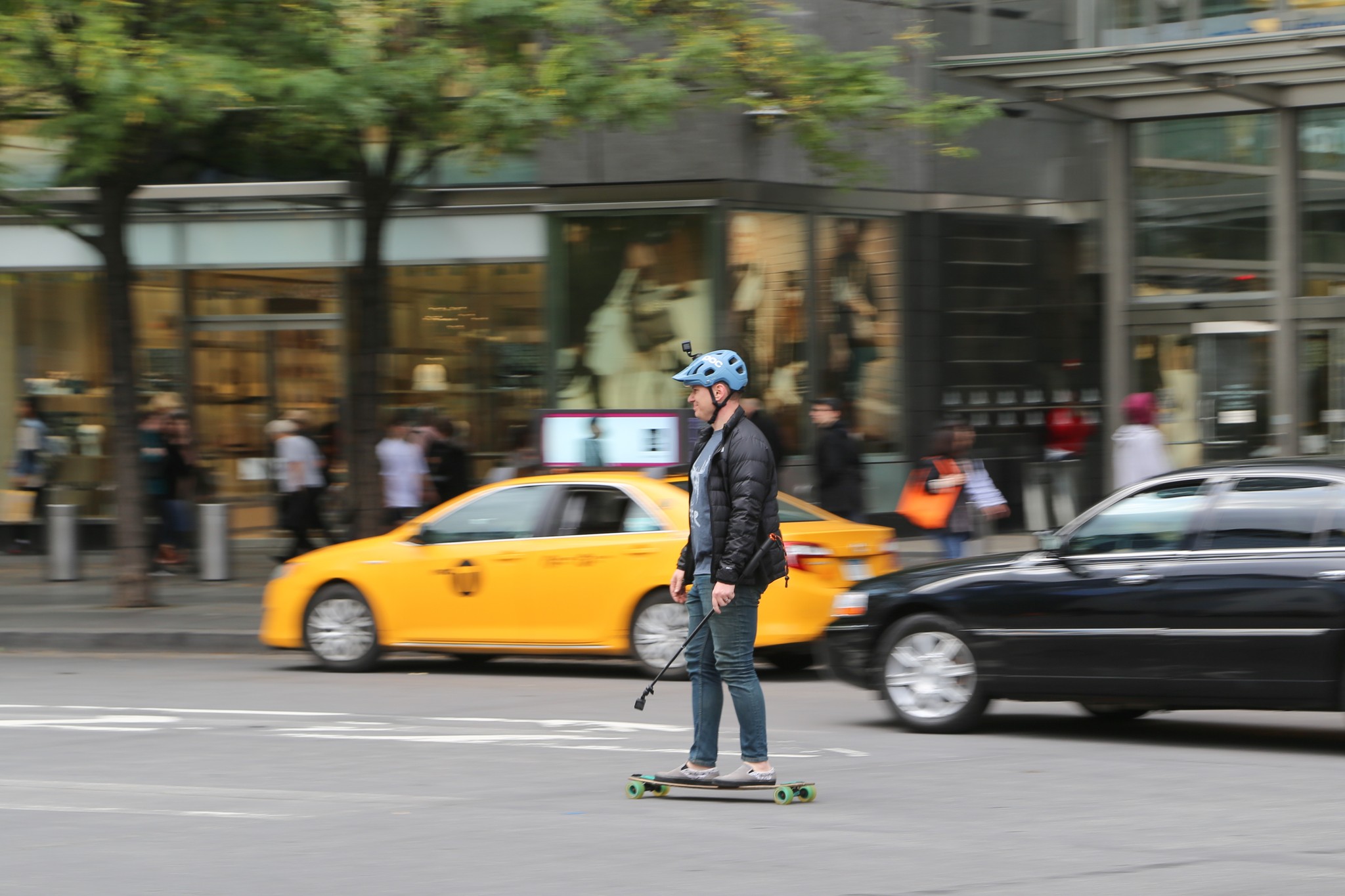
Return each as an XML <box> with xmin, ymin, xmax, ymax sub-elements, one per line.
<box><xmin>1123</xmin><ymin>392</ymin><xmax>1155</xmax><ymax>423</ymax></box>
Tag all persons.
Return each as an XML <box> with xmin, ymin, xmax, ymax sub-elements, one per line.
<box><xmin>137</xmin><ymin>409</ymin><xmax>213</xmax><ymax>580</ymax></box>
<box><xmin>911</xmin><ymin>397</ymin><xmax>1006</xmax><ymax>563</ymax></box>
<box><xmin>806</xmin><ymin>394</ymin><xmax>866</xmax><ymax>522</ymax></box>
<box><xmin>654</xmin><ymin>350</ymin><xmax>790</xmax><ymax>792</ymax></box>
<box><xmin>261</xmin><ymin>408</ymin><xmax>475</xmax><ymax>572</ymax></box>
<box><xmin>581</xmin><ymin>246</ymin><xmax>713</xmax><ymax>410</ymax></box>
<box><xmin>802</xmin><ymin>213</ymin><xmax>885</xmax><ymax>443</ymax></box>
<box><xmin>1112</xmin><ymin>403</ymin><xmax>1173</xmax><ymax>496</ymax></box>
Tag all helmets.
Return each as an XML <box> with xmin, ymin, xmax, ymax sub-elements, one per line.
<box><xmin>672</xmin><ymin>349</ymin><xmax>748</xmax><ymax>392</ymax></box>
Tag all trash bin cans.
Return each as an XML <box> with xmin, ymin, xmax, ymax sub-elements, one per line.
<box><xmin>45</xmin><ymin>504</ymin><xmax>81</xmax><ymax>580</ymax></box>
<box><xmin>197</xmin><ymin>503</ymin><xmax>232</xmax><ymax>580</ymax></box>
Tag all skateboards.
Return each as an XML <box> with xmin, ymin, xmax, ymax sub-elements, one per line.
<box><xmin>627</xmin><ymin>774</ymin><xmax>816</xmax><ymax>804</ymax></box>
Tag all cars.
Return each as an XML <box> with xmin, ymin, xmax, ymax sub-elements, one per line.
<box><xmin>808</xmin><ymin>453</ymin><xmax>1344</xmax><ymax>732</ymax></box>
<box><xmin>252</xmin><ymin>454</ymin><xmax>904</xmax><ymax>677</ymax></box>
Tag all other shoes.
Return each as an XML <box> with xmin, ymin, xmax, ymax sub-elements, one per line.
<box><xmin>654</xmin><ymin>760</ymin><xmax>720</xmax><ymax>785</ymax></box>
<box><xmin>713</xmin><ymin>762</ymin><xmax>778</xmax><ymax>786</ymax></box>
